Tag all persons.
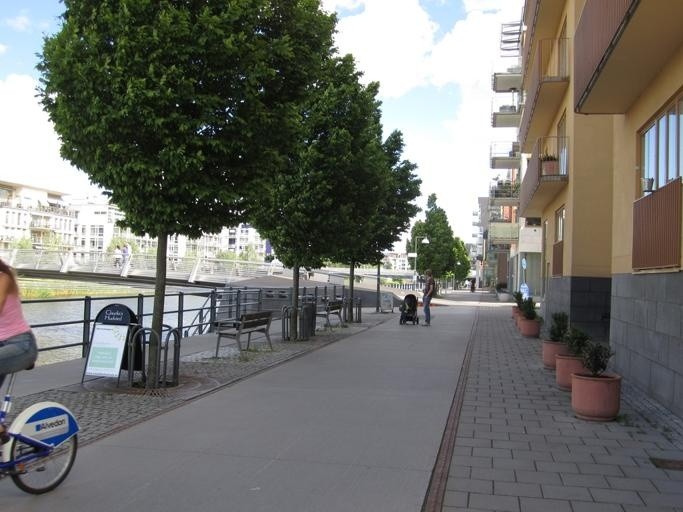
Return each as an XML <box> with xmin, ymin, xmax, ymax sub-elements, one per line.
<box><xmin>0</xmin><ymin>258</ymin><xmax>37</xmax><ymax>444</ymax></box>
<box><xmin>121</xmin><ymin>245</ymin><xmax>128</xmax><ymax>267</ymax></box>
<box><xmin>113</xmin><ymin>245</ymin><xmax>122</xmax><ymax>269</ymax></box>
<box><xmin>422</xmin><ymin>271</ymin><xmax>435</xmax><ymax>326</ymax></box>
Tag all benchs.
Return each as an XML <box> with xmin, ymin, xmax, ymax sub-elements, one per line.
<box><xmin>216</xmin><ymin>311</ymin><xmax>273</xmax><ymax>361</ymax></box>
<box><xmin>315</xmin><ymin>296</ymin><xmax>343</xmax><ymax>334</ymax></box>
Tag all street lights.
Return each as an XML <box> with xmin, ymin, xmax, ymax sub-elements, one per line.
<box><xmin>413</xmin><ymin>234</ymin><xmax>429</xmax><ymax>282</ymax></box>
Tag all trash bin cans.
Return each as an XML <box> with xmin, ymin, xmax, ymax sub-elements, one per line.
<box><xmin>298</xmin><ymin>295</ymin><xmax>316</xmax><ymax>336</ymax></box>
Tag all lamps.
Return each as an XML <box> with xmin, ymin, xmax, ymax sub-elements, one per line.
<box><xmin>641</xmin><ymin>177</ymin><xmax>657</xmax><ymax>194</ymax></box>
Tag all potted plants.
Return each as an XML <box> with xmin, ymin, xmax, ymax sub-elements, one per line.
<box><xmin>541</xmin><ymin>146</ymin><xmax>559</xmax><ymax>176</ymax></box>
<box><xmin>512</xmin><ymin>291</ymin><xmax>623</xmax><ymax>421</ymax></box>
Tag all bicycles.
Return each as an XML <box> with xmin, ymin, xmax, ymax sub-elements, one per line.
<box><xmin>0</xmin><ymin>363</ymin><xmax>79</xmax><ymax>493</ymax></box>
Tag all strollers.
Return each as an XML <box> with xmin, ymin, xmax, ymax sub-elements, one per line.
<box><xmin>400</xmin><ymin>294</ymin><xmax>419</xmax><ymax>326</ymax></box>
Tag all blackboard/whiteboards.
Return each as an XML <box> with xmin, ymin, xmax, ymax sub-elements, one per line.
<box><xmin>381</xmin><ymin>292</ymin><xmax>393</xmax><ymax>310</ymax></box>
<box><xmin>83</xmin><ymin>304</ymin><xmax>130</xmax><ymax>377</ymax></box>
